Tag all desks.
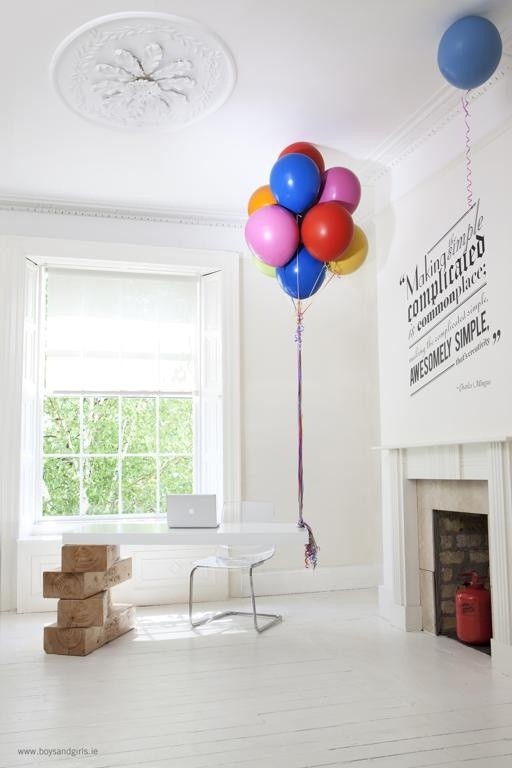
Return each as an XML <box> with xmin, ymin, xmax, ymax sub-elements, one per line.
<box><xmin>63</xmin><ymin>522</ymin><xmax>310</xmax><ymax>546</ymax></box>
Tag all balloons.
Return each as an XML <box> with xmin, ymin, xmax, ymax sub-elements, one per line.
<box><xmin>435</xmin><ymin>14</ymin><xmax>503</xmax><ymax>91</ymax></box>
<box><xmin>326</xmin><ymin>223</ymin><xmax>369</xmax><ymax>276</ymax></box>
<box><xmin>277</xmin><ymin>141</ymin><xmax>325</xmax><ymax>176</ymax></box>
<box><xmin>313</xmin><ymin>166</ymin><xmax>362</xmax><ymax>215</ymax></box>
<box><xmin>300</xmin><ymin>201</ymin><xmax>354</xmax><ymax>263</ymax></box>
<box><xmin>252</xmin><ymin>253</ymin><xmax>276</xmax><ymax>278</ymax></box>
<box><xmin>247</xmin><ymin>185</ymin><xmax>278</xmax><ymax>217</ymax></box>
<box><xmin>274</xmin><ymin>243</ymin><xmax>326</xmax><ymax>300</ymax></box>
<box><xmin>244</xmin><ymin>204</ymin><xmax>300</xmax><ymax>268</ymax></box>
<box><xmin>269</xmin><ymin>152</ymin><xmax>322</xmax><ymax>217</ymax></box>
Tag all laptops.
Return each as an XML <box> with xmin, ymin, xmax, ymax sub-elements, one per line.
<box><xmin>167</xmin><ymin>494</ymin><xmax>219</xmax><ymax>529</ymax></box>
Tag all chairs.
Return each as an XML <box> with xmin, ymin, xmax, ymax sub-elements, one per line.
<box><xmin>189</xmin><ymin>501</ymin><xmax>283</xmax><ymax>633</ymax></box>
